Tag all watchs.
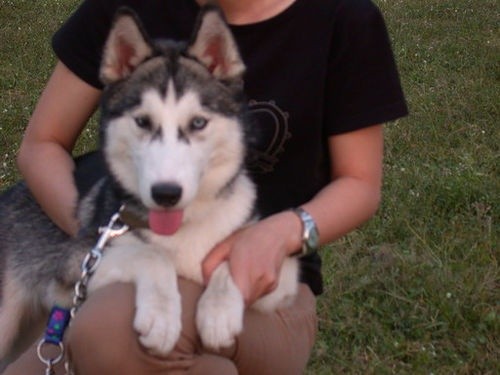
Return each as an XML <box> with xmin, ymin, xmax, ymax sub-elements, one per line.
<box><xmin>284</xmin><ymin>207</ymin><xmax>323</xmax><ymax>258</ymax></box>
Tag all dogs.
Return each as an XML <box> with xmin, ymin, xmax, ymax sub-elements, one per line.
<box><xmin>0</xmin><ymin>0</ymin><xmax>300</xmax><ymax>364</ymax></box>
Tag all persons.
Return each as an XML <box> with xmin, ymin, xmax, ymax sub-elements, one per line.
<box><xmin>5</xmin><ymin>0</ymin><xmax>410</xmax><ymax>375</ymax></box>
